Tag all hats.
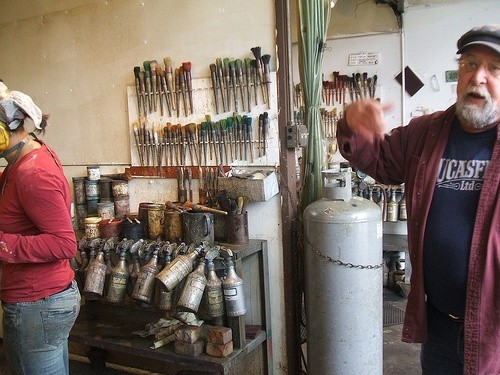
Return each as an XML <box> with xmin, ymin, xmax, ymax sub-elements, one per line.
<box><xmin>456</xmin><ymin>25</ymin><xmax>500</xmax><ymax>54</ymax></box>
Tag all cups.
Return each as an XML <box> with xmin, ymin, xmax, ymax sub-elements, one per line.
<box><xmin>98</xmin><ymin>219</ymin><xmax>122</xmax><ymax>238</ymax></box>
<box><xmin>138</xmin><ymin>202</ymin><xmax>249</xmax><ymax>247</ymax></box>
<box><xmin>120</xmin><ymin>220</ymin><xmax>144</xmax><ymax>241</ymax></box>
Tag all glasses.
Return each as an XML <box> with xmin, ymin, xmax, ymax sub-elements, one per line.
<box><xmin>458</xmin><ymin>58</ymin><xmax>500</xmax><ymax>77</ymax></box>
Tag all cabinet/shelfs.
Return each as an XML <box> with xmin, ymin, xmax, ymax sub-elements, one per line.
<box><xmin>67</xmin><ymin>239</ymin><xmax>270</xmax><ymax>375</ymax></box>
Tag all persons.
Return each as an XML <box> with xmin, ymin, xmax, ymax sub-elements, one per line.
<box><xmin>336</xmin><ymin>24</ymin><xmax>500</xmax><ymax>375</ymax></box>
<box><xmin>0</xmin><ymin>81</ymin><xmax>80</xmax><ymax>375</ymax></box>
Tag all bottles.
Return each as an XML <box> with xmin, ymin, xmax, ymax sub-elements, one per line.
<box><xmin>355</xmin><ymin>188</ymin><xmax>407</xmax><ymax>222</ymax></box>
<box><xmin>70</xmin><ymin>167</ymin><xmax>129</xmax><ymax>238</ymax></box>
<box><xmin>69</xmin><ymin>248</ymin><xmax>247</xmax><ymax>319</ymax></box>
